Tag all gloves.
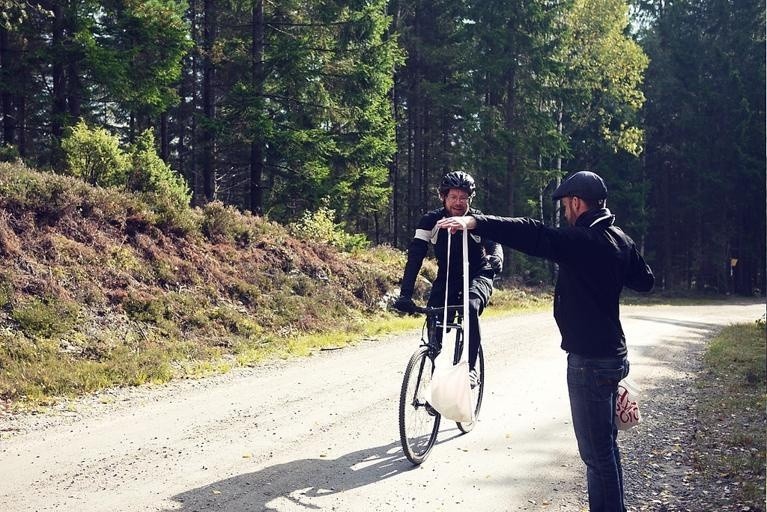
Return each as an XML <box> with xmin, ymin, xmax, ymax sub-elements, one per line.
<box><xmin>396</xmin><ymin>294</ymin><xmax>416</xmax><ymax>316</ymax></box>
<box><xmin>486</xmin><ymin>255</ymin><xmax>502</xmax><ymax>274</ymax></box>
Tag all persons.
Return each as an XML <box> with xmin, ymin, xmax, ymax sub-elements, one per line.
<box><xmin>435</xmin><ymin>171</ymin><xmax>656</xmax><ymax>511</ymax></box>
<box><xmin>393</xmin><ymin>168</ymin><xmax>506</xmax><ymax>390</ymax></box>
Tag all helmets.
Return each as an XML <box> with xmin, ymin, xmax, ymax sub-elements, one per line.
<box><xmin>439</xmin><ymin>170</ymin><xmax>476</xmax><ymax>195</ymax></box>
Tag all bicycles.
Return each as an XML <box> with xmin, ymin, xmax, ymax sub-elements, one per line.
<box><xmin>393</xmin><ymin>300</ymin><xmax>486</xmax><ymax>466</ymax></box>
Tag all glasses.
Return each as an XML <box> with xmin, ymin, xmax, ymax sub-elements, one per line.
<box><xmin>443</xmin><ymin>194</ymin><xmax>471</xmax><ymax>202</ymax></box>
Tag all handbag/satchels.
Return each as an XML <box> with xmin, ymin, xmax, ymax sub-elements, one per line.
<box><xmin>423</xmin><ymin>352</ymin><xmax>474</xmax><ymax>423</ymax></box>
<box><xmin>615</xmin><ymin>376</ymin><xmax>643</xmax><ymax>431</ymax></box>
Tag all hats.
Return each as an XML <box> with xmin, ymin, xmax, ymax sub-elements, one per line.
<box><xmin>551</xmin><ymin>170</ymin><xmax>608</xmax><ymax>201</ymax></box>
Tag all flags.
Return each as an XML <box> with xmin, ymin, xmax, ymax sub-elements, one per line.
<box><xmin>731</xmin><ymin>259</ymin><xmax>739</xmax><ymax>267</ymax></box>
